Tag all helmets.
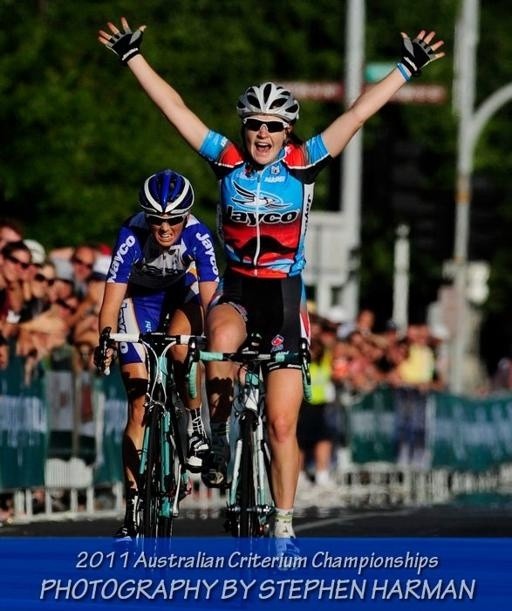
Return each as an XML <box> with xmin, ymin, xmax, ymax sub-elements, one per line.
<box><xmin>138</xmin><ymin>169</ymin><xmax>196</xmax><ymax>216</ymax></box>
<box><xmin>235</xmin><ymin>82</ymin><xmax>301</xmax><ymax>123</ymax></box>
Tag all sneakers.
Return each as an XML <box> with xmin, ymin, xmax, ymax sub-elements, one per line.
<box><xmin>270</xmin><ymin>512</ymin><xmax>304</xmax><ymax>573</ymax></box>
<box><xmin>185</xmin><ymin>404</ymin><xmax>231</xmax><ymax>487</ymax></box>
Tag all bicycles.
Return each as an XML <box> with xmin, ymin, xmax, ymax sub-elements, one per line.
<box><xmin>186</xmin><ymin>336</ymin><xmax>312</xmax><ymax>587</ymax></box>
<box><xmin>95</xmin><ymin>325</ymin><xmax>206</xmax><ymax>572</ymax></box>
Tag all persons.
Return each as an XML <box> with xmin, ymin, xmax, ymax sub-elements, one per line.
<box><xmin>97</xmin><ymin>17</ymin><xmax>446</xmax><ymax>570</ymax></box>
<box><xmin>0</xmin><ymin>224</ymin><xmax>510</xmax><ymax>525</ymax></box>
<box><xmin>93</xmin><ymin>168</ymin><xmax>223</xmax><ymax>549</ymax></box>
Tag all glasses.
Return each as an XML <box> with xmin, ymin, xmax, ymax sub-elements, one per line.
<box><xmin>144</xmin><ymin>211</ymin><xmax>192</xmax><ymax>227</ymax></box>
<box><xmin>240</xmin><ymin>117</ymin><xmax>290</xmax><ymax>133</ymax></box>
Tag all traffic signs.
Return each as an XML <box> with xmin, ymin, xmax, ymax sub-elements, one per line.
<box><xmin>365</xmin><ymin>62</ymin><xmax>396</xmax><ymax>83</ymax></box>
<box><xmin>276</xmin><ymin>81</ymin><xmax>342</xmax><ymax>100</ymax></box>
<box><xmin>363</xmin><ymin>86</ymin><xmax>443</xmax><ymax>105</ymax></box>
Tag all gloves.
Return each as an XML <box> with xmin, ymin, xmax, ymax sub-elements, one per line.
<box><xmin>104</xmin><ymin>26</ymin><xmax>143</xmax><ymax>65</ymax></box>
<box><xmin>401</xmin><ymin>37</ymin><xmax>436</xmax><ymax>76</ymax></box>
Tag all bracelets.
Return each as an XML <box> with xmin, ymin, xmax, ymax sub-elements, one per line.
<box><xmin>396</xmin><ymin>61</ymin><xmax>410</xmax><ymax>83</ymax></box>
